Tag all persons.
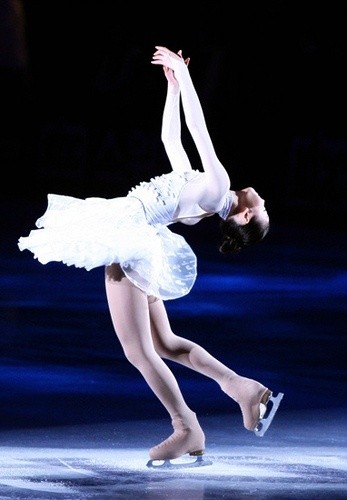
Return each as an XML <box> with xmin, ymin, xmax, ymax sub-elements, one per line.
<box><xmin>104</xmin><ymin>46</ymin><xmax>283</xmax><ymax>470</ymax></box>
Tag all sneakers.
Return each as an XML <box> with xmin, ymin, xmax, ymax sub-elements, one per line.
<box><xmin>145</xmin><ymin>410</ymin><xmax>205</xmax><ymax>468</ymax></box>
<box><xmin>219</xmin><ymin>375</ymin><xmax>283</xmax><ymax>436</ymax></box>
